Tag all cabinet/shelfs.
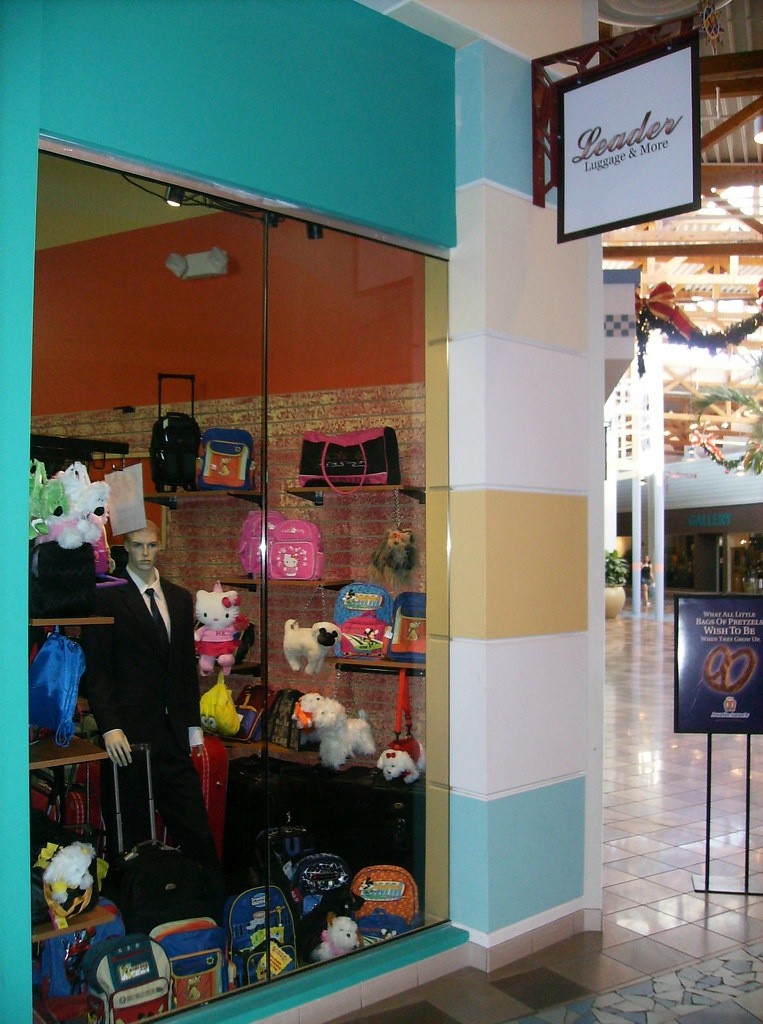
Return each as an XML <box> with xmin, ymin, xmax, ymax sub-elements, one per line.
<box><xmin>30</xmin><ymin>483</ymin><xmax>427</xmax><ymax>945</ymax></box>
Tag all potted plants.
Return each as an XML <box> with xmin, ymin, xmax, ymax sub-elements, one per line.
<box><xmin>604</xmin><ymin>549</ymin><xmax>628</xmax><ymax>621</ymax></box>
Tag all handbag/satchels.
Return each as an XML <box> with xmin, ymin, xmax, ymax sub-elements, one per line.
<box><xmin>298</xmin><ymin>426</ymin><xmax>401</xmax><ymax>494</ymax></box>
<box><xmin>267</xmin><ymin>687</ymin><xmax>320</xmax><ymax>752</ymax></box>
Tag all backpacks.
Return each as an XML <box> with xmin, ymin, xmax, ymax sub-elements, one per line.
<box><xmin>198</xmin><ymin>428</ymin><xmax>258</xmax><ymax>490</ymax></box>
<box><xmin>81</xmin><ymin>852</ymin><xmax>420</xmax><ymax>1024</ymax></box>
<box><xmin>29</xmin><ymin>626</ymin><xmax>86</xmax><ymax>748</ymax></box>
<box><xmin>32</xmin><ymin>895</ymin><xmax>125</xmax><ymax>996</ymax></box>
<box><xmin>29</xmin><ymin>538</ymin><xmax>96</xmax><ymax>618</ymax></box>
<box><xmin>386</xmin><ymin>592</ymin><xmax>426</xmax><ymax>663</ymax></box>
<box><xmin>269</xmin><ymin>519</ymin><xmax>325</xmax><ymax>581</ymax></box>
<box><xmin>215</xmin><ymin>684</ymin><xmax>277</xmax><ymax>744</ymax></box>
<box><xmin>238</xmin><ymin>510</ymin><xmax>285</xmax><ymax>579</ymax></box>
<box><xmin>334</xmin><ymin>583</ymin><xmax>393</xmax><ymax>660</ymax></box>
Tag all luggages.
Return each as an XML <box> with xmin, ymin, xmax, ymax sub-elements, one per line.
<box><xmin>29</xmin><ymin>764</ymin><xmax>99</xmax><ymax>846</ymax></box>
<box><xmin>99</xmin><ymin>742</ymin><xmax>221</xmax><ymax>935</ymax></box>
<box><xmin>240</xmin><ymin>807</ymin><xmax>322</xmax><ymax>890</ymax></box>
<box><xmin>149</xmin><ymin>372</ymin><xmax>202</xmax><ymax>493</ymax></box>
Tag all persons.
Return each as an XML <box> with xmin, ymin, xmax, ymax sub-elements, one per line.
<box><xmin>641</xmin><ymin>555</ymin><xmax>654</xmax><ymax>607</ymax></box>
<box><xmin>82</xmin><ymin>519</ymin><xmax>221</xmax><ymax>937</ymax></box>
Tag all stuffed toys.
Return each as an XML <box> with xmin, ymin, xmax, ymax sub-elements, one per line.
<box><xmin>194</xmin><ymin>580</ymin><xmax>250</xmax><ymax>671</ymax></box>
<box><xmin>283</xmin><ymin>619</ymin><xmax>342</xmax><ymax>676</ymax></box>
<box><xmin>28</xmin><ymin>458</ymin><xmax>110</xmax><ymax>550</ymax></box>
<box><xmin>376</xmin><ymin>739</ymin><xmax>426</xmax><ymax>784</ymax></box>
<box><xmin>312</xmin><ymin>916</ymin><xmax>357</xmax><ymax>959</ymax></box>
<box><xmin>42</xmin><ymin>840</ymin><xmax>96</xmax><ymax>904</ymax></box>
<box><xmin>292</xmin><ymin>692</ymin><xmax>377</xmax><ymax>769</ymax></box>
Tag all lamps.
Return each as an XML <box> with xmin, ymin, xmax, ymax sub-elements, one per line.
<box><xmin>166</xmin><ymin>248</ymin><xmax>228</xmax><ymax>284</ymax></box>
<box><xmin>164</xmin><ymin>183</ymin><xmax>185</xmax><ymax>207</ymax></box>
<box><xmin>306</xmin><ymin>222</ymin><xmax>324</xmax><ymax>241</ymax></box>
<box><xmin>260</xmin><ymin>211</ymin><xmax>279</xmax><ymax>225</ymax></box>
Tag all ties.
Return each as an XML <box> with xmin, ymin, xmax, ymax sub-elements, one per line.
<box><xmin>145</xmin><ymin>588</ymin><xmax>169</xmax><ymax>643</ymax></box>
